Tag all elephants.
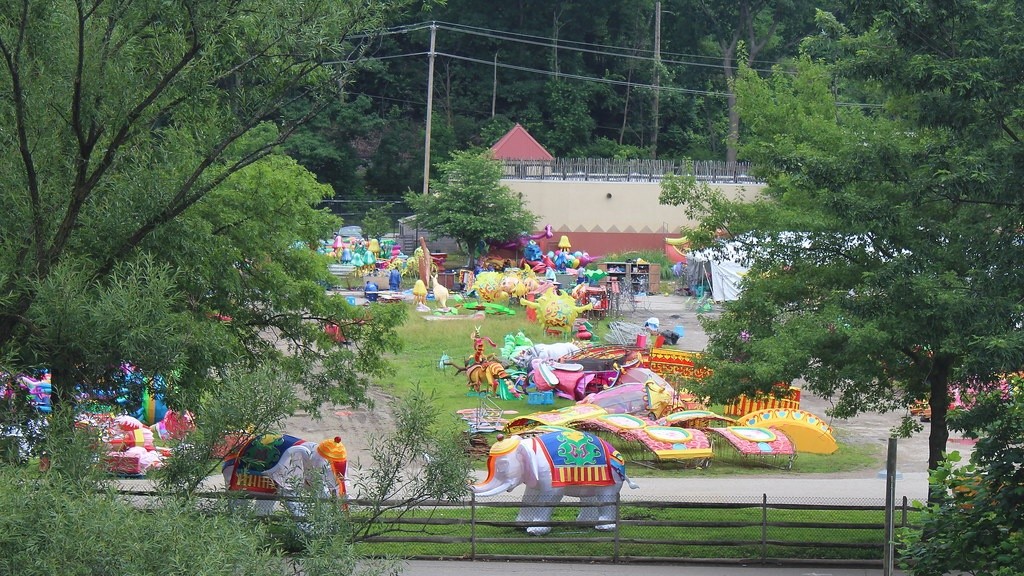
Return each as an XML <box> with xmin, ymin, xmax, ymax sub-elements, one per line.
<box><xmin>464</xmin><ymin>430</ymin><xmax>640</xmax><ymax>536</ymax></box>
<box><xmin>221</xmin><ymin>433</ymin><xmax>355</xmax><ymax>534</ymax></box>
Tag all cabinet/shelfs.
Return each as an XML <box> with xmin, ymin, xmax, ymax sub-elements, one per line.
<box><xmin>596</xmin><ymin>260</ymin><xmax>651</xmax><ymax>296</ymax></box>
